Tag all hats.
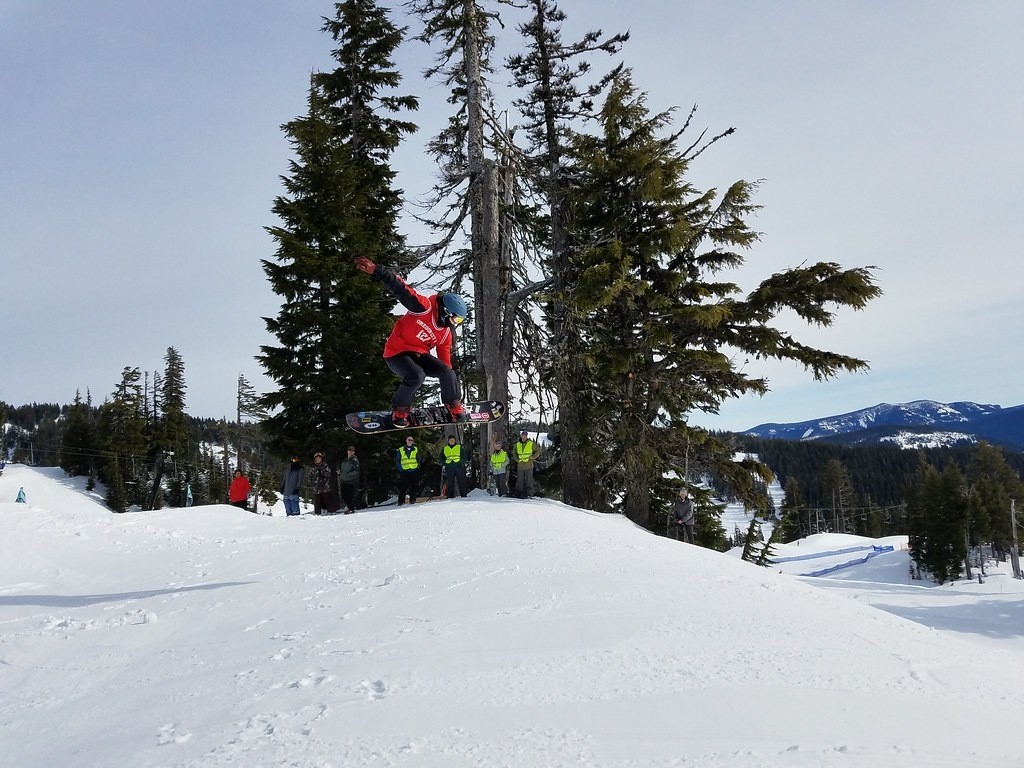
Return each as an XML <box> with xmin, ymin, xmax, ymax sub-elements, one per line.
<box><xmin>348</xmin><ymin>446</ymin><xmax>354</xmax><ymax>450</ymax></box>
<box><xmin>448</xmin><ymin>434</ymin><xmax>456</xmax><ymax>441</ymax></box>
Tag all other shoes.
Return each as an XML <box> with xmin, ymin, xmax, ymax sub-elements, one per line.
<box><xmin>344</xmin><ymin>509</ymin><xmax>356</xmax><ymax>514</ymax></box>
<box><xmin>410</xmin><ymin>501</ymin><xmax>416</xmax><ymax>504</ymax></box>
<box><xmin>397</xmin><ymin>502</ymin><xmax>403</xmax><ymax>505</ymax></box>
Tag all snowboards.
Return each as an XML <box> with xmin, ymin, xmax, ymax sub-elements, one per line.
<box><xmin>345</xmin><ymin>399</ymin><xmax>506</xmax><ymax>435</ymax></box>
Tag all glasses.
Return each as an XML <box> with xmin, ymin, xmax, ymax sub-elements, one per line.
<box><xmin>450</xmin><ymin>316</ymin><xmax>464</xmax><ymax>327</ymax></box>
<box><xmin>408</xmin><ymin>439</ymin><xmax>413</xmax><ymax>441</ymax></box>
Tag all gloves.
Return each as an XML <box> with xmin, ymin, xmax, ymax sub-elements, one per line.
<box><xmin>354</xmin><ymin>255</ymin><xmax>376</xmax><ymax>274</ymax></box>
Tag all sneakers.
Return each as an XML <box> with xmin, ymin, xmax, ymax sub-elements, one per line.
<box><xmin>391</xmin><ymin>405</ymin><xmax>411</xmax><ymax>428</ymax></box>
<box><xmin>445</xmin><ymin>399</ymin><xmax>467</xmax><ymax>422</ymax></box>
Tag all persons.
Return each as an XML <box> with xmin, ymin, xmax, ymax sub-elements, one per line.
<box><xmin>339</xmin><ymin>445</ymin><xmax>361</xmax><ymax>514</ymax></box>
<box><xmin>354</xmin><ymin>256</ymin><xmax>467</xmax><ymax>428</ymax></box>
<box><xmin>15</xmin><ymin>487</ymin><xmax>26</xmax><ymax>503</ymax></box>
<box><xmin>280</xmin><ymin>453</ymin><xmax>305</xmax><ymax>516</ymax></box>
<box><xmin>439</xmin><ymin>434</ymin><xmax>468</xmax><ymax>499</ymax></box>
<box><xmin>396</xmin><ymin>435</ymin><xmax>421</xmax><ymax>506</ymax></box>
<box><xmin>306</xmin><ymin>453</ymin><xmax>333</xmax><ymax>515</ymax></box>
<box><xmin>672</xmin><ymin>487</ymin><xmax>695</xmax><ymax>545</ymax></box>
<box><xmin>229</xmin><ymin>469</ymin><xmax>250</xmax><ymax>510</ymax></box>
<box><xmin>490</xmin><ymin>440</ymin><xmax>510</xmax><ymax>497</ymax></box>
<box><xmin>513</xmin><ymin>431</ymin><xmax>540</xmax><ymax>499</ymax></box>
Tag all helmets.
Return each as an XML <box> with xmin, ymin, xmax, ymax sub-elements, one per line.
<box><xmin>443</xmin><ymin>291</ymin><xmax>468</xmax><ymax>316</ymax></box>
